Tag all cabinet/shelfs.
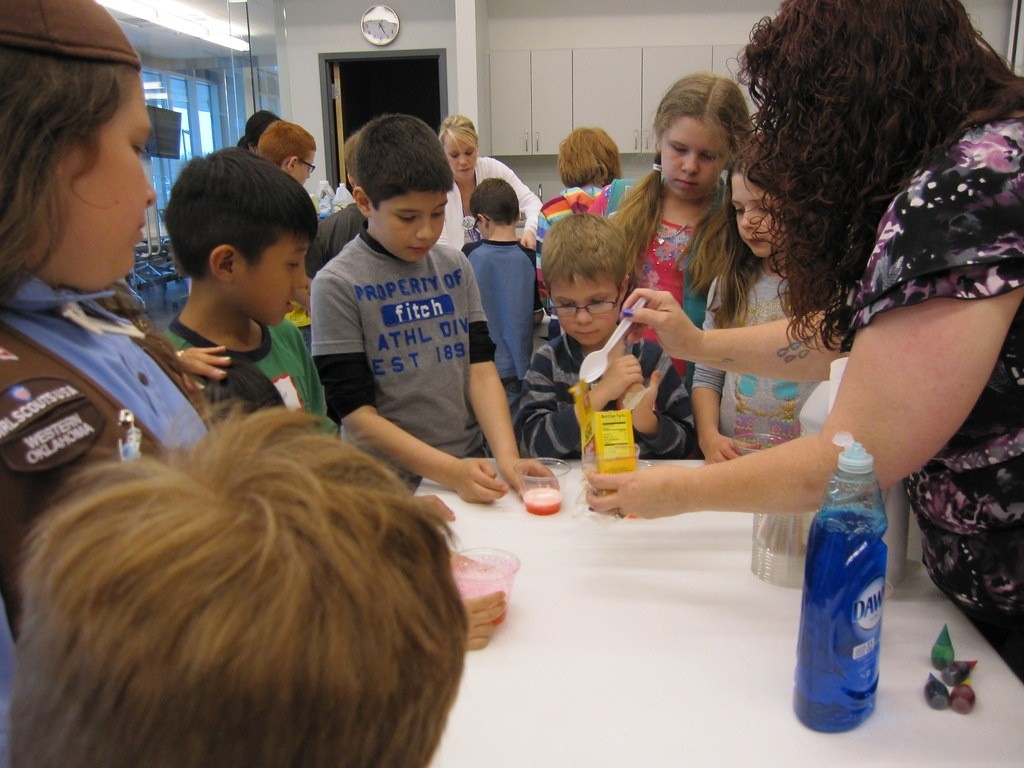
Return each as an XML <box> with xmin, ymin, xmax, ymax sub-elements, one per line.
<box><xmin>486</xmin><ymin>40</ymin><xmax>759</xmax><ymax>154</ymax></box>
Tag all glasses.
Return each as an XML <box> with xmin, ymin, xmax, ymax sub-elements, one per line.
<box><xmin>546</xmin><ymin>282</ymin><xmax>627</xmax><ymax>318</ymax></box>
<box><xmin>284</xmin><ymin>157</ymin><xmax>315</xmax><ymax>173</ymax></box>
<box><xmin>472</xmin><ymin>213</ymin><xmax>491</xmax><ymax>235</ymax></box>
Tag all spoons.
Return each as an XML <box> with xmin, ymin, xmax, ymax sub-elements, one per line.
<box><xmin>579</xmin><ymin>297</ymin><xmax>646</xmax><ymax>383</ymax></box>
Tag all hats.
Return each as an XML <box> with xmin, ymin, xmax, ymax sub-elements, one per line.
<box><xmin>0</xmin><ymin>0</ymin><xmax>142</xmax><ymax>71</ymax></box>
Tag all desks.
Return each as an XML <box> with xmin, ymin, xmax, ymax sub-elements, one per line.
<box><xmin>407</xmin><ymin>457</ymin><xmax>1024</xmax><ymax>768</ymax></box>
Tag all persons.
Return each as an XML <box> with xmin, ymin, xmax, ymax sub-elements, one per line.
<box><xmin>612</xmin><ymin>74</ymin><xmax>752</xmax><ymax>390</ymax></box>
<box><xmin>690</xmin><ymin>130</ymin><xmax>839</xmax><ymax>465</ymax></box>
<box><xmin>7</xmin><ymin>404</ymin><xmax>469</xmax><ymax>768</ymax></box>
<box><xmin>158</xmin><ymin>105</ymin><xmax>694</xmax><ymax>492</ymax></box>
<box><xmin>0</xmin><ymin>0</ymin><xmax>511</xmax><ymax>651</ymax></box>
<box><xmin>310</xmin><ymin>116</ymin><xmax>561</xmax><ymax>507</ymax></box>
<box><xmin>587</xmin><ymin>0</ymin><xmax>1024</xmax><ymax>675</ymax></box>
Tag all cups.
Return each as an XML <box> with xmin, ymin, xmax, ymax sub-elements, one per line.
<box><xmin>515</xmin><ymin>457</ymin><xmax>571</xmax><ymax>516</ymax></box>
<box><xmin>455</xmin><ymin>547</ymin><xmax>520</xmax><ymax>624</ymax></box>
<box><xmin>752</xmin><ymin>512</ymin><xmax>815</xmax><ymax>589</ymax></box>
<box><xmin>733</xmin><ymin>432</ymin><xmax>787</xmax><ymax>455</ymax></box>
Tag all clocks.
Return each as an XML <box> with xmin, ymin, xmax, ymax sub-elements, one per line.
<box><xmin>359</xmin><ymin>5</ymin><xmax>400</xmax><ymax>45</ymax></box>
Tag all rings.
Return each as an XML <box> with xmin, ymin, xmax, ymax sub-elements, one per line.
<box><xmin>616</xmin><ymin>509</ymin><xmax>623</xmax><ymax>520</ymax></box>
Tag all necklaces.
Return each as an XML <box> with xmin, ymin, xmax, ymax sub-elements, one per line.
<box><xmin>654</xmin><ymin>204</ymin><xmax>704</xmax><ymax>246</ymax></box>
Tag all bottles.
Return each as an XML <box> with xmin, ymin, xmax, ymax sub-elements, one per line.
<box><xmin>318</xmin><ymin>181</ymin><xmax>335</xmax><ymax>222</ymax></box>
<box><xmin>335</xmin><ymin>183</ymin><xmax>356</xmax><ymax>212</ymax></box>
<box><xmin>799</xmin><ymin>357</ymin><xmax>909</xmax><ymax>586</ymax></box>
<box><xmin>793</xmin><ymin>431</ymin><xmax>888</xmax><ymax>733</ymax></box>
